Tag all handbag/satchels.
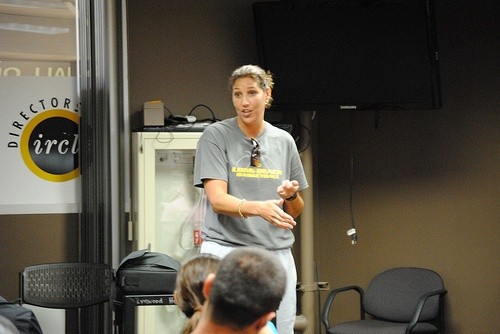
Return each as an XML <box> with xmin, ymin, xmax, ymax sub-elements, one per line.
<box><xmin>114</xmin><ymin>250</ymin><xmax>181</xmax><ymax>294</ymax></box>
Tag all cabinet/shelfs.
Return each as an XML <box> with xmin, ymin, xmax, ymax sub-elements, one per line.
<box><xmin>132</xmin><ymin>132</ymin><xmax>204</xmax><ymax>265</ymax></box>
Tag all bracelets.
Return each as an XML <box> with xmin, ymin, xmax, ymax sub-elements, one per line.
<box><xmin>238</xmin><ymin>198</ymin><xmax>249</xmax><ymax>219</ymax></box>
<box><xmin>285</xmin><ymin>191</ymin><xmax>297</xmax><ymax>201</ymax></box>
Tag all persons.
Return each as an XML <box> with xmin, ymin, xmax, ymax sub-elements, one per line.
<box><xmin>173</xmin><ymin>65</ymin><xmax>310</xmax><ymax>334</ymax></box>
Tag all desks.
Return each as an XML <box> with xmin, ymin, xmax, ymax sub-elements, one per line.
<box><xmin>123</xmin><ymin>295</ymin><xmax>180</xmax><ymax>334</ymax></box>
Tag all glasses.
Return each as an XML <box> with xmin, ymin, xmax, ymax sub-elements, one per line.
<box><xmin>250</xmin><ymin>139</ymin><xmax>262</xmax><ymax>167</ymax></box>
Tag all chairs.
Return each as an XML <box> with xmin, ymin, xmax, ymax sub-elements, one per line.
<box><xmin>321</xmin><ymin>268</ymin><xmax>448</xmax><ymax>334</ymax></box>
<box><xmin>19</xmin><ymin>263</ymin><xmax>112</xmax><ymax>334</ymax></box>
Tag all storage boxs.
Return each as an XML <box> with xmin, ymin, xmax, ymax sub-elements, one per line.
<box><xmin>143</xmin><ymin>103</ymin><xmax>165</xmax><ymax>126</ymax></box>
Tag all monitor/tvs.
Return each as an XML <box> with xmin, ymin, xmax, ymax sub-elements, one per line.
<box><xmin>252</xmin><ymin>0</ymin><xmax>443</xmax><ymax>113</ymax></box>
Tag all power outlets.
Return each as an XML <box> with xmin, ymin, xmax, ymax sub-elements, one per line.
<box><xmin>296</xmin><ymin>282</ymin><xmax>330</xmax><ymax>291</ymax></box>
